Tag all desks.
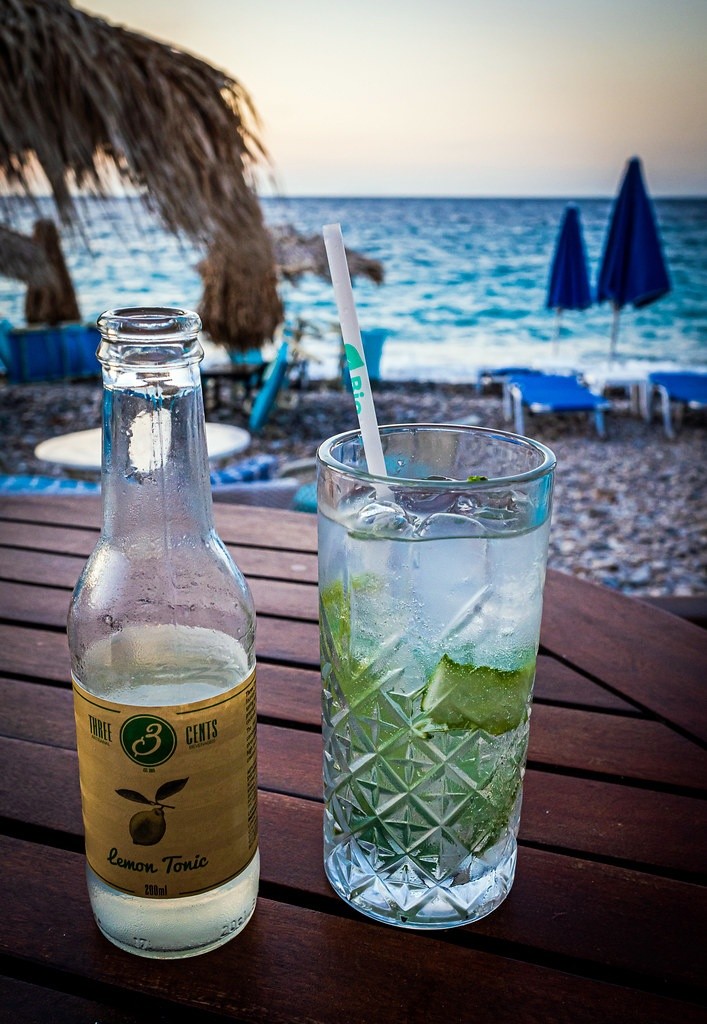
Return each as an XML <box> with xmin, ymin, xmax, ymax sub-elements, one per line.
<box><xmin>199</xmin><ymin>364</ymin><xmax>269</xmax><ymax>421</ymax></box>
<box><xmin>0</xmin><ymin>491</ymin><xmax>707</xmax><ymax>1024</ymax></box>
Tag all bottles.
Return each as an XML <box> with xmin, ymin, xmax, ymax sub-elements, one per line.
<box><xmin>67</xmin><ymin>306</ymin><xmax>261</xmax><ymax>964</ymax></box>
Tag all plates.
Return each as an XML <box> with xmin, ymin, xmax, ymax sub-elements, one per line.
<box><xmin>33</xmin><ymin>417</ymin><xmax>250</xmax><ymax>467</ymax></box>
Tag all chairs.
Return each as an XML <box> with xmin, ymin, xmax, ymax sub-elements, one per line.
<box><xmin>649</xmin><ymin>371</ymin><xmax>707</xmax><ymax>441</ymax></box>
<box><xmin>502</xmin><ymin>373</ymin><xmax>612</xmax><ymax>442</ymax></box>
<box><xmin>337</xmin><ymin>328</ymin><xmax>393</xmax><ymax>394</ymax></box>
<box><xmin>226</xmin><ymin>350</ymin><xmax>309</xmax><ymax>394</ymax></box>
<box><xmin>474</xmin><ymin>365</ymin><xmax>533</xmax><ymax>394</ymax></box>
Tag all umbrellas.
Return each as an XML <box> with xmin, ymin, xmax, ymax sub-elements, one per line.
<box><xmin>190</xmin><ymin>223</ymin><xmax>386</xmax><ymax>286</ymax></box>
<box><xmin>544</xmin><ymin>205</ymin><xmax>591</xmax><ymax>351</ymax></box>
<box><xmin>22</xmin><ymin>218</ymin><xmax>83</xmax><ymax>328</ymax></box>
<box><xmin>0</xmin><ymin>0</ymin><xmax>267</xmax><ymax>256</ymax></box>
<box><xmin>594</xmin><ymin>155</ymin><xmax>674</xmax><ymax>367</ymax></box>
<box><xmin>0</xmin><ymin>229</ymin><xmax>61</xmax><ymax>295</ymax></box>
<box><xmin>197</xmin><ymin>194</ymin><xmax>286</xmax><ymax>349</ymax></box>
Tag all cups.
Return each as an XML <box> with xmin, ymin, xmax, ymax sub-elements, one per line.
<box><xmin>315</xmin><ymin>424</ymin><xmax>557</xmax><ymax>932</ymax></box>
